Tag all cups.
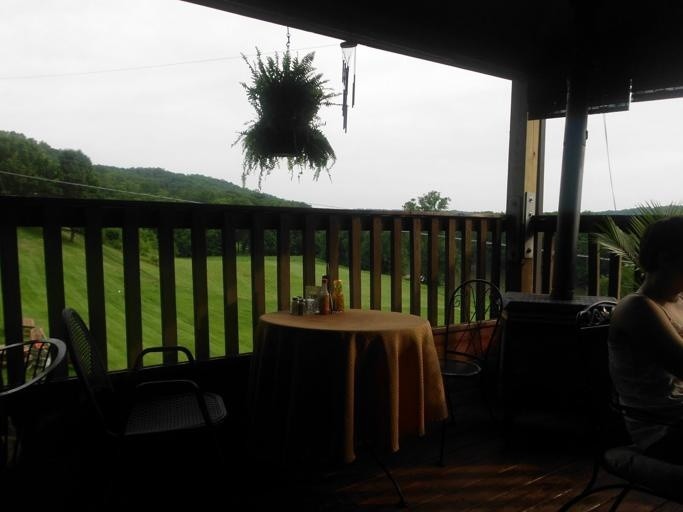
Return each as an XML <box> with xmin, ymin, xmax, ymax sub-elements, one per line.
<box><xmin>305</xmin><ymin>299</ymin><xmax>317</xmax><ymax>315</ymax></box>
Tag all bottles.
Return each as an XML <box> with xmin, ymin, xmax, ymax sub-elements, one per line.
<box><xmin>292</xmin><ymin>296</ymin><xmax>304</xmax><ymax>316</ymax></box>
<box><xmin>319</xmin><ymin>274</ymin><xmax>344</xmax><ymax>315</ymax></box>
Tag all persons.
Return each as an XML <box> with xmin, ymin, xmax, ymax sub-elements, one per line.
<box><xmin>608</xmin><ymin>214</ymin><xmax>683</xmax><ymax>467</ymax></box>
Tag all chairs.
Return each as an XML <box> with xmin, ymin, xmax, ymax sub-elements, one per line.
<box><xmin>0</xmin><ymin>308</ymin><xmax>229</xmax><ymax>469</ymax></box>
<box><xmin>439</xmin><ymin>280</ymin><xmax>503</xmax><ymax>465</ymax></box>
<box><xmin>559</xmin><ymin>297</ymin><xmax>682</xmax><ymax>511</ymax></box>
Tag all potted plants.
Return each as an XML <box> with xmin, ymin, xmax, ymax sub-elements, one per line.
<box><xmin>244</xmin><ymin>58</ymin><xmax>334</xmax><ymax>168</ymax></box>
